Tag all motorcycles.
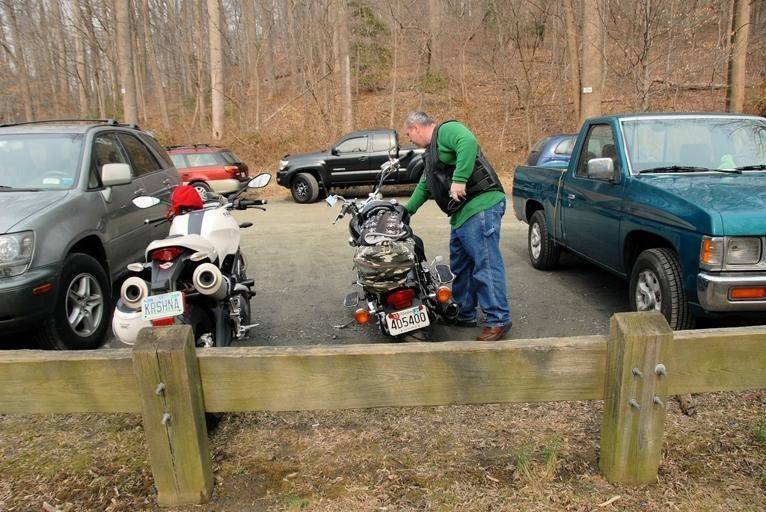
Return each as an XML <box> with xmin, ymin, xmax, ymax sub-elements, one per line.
<box><xmin>325</xmin><ymin>146</ymin><xmax>462</xmax><ymax>342</ymax></box>
<box><xmin>121</xmin><ymin>173</ymin><xmax>272</xmax><ymax>348</ymax></box>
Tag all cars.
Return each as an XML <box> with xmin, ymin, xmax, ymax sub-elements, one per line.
<box><xmin>527</xmin><ymin>134</ymin><xmax>601</xmax><ymax>168</ymax></box>
<box><xmin>0</xmin><ymin>120</ymin><xmax>182</xmax><ymax>350</ymax></box>
<box><xmin>166</xmin><ymin>144</ymin><xmax>250</xmax><ymax>202</ymax></box>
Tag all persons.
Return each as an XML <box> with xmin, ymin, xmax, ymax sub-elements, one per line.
<box><xmin>402</xmin><ymin>111</ymin><xmax>512</xmax><ymax>341</ymax></box>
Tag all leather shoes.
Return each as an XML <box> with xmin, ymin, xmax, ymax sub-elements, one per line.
<box><xmin>445</xmin><ymin>317</ymin><xmax>478</xmax><ymax>328</ymax></box>
<box><xmin>477</xmin><ymin>321</ymin><xmax>511</xmax><ymax>341</ymax></box>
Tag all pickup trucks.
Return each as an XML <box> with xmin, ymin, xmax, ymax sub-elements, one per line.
<box><xmin>276</xmin><ymin>128</ymin><xmax>434</xmax><ymax>203</ymax></box>
<box><xmin>513</xmin><ymin>112</ymin><xmax>766</xmax><ymax>330</ymax></box>
